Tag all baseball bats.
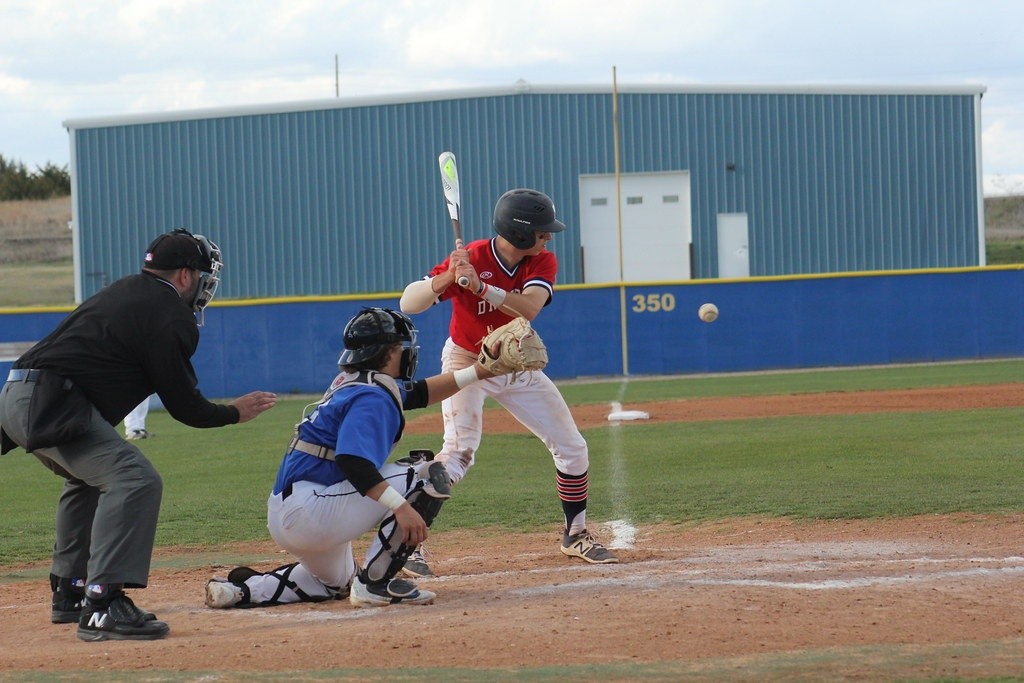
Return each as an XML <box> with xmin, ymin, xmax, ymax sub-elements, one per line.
<box><xmin>438</xmin><ymin>151</ymin><xmax>470</xmax><ymax>288</ymax></box>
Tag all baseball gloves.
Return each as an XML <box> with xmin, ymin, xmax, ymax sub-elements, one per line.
<box><xmin>477</xmin><ymin>315</ymin><xmax>549</xmax><ymax>377</ymax></box>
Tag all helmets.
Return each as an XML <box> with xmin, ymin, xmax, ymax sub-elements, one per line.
<box><xmin>337</xmin><ymin>305</ymin><xmax>420</xmax><ymax>381</ymax></box>
<box><xmin>493</xmin><ymin>188</ymin><xmax>567</xmax><ymax>250</ymax></box>
<box><xmin>144</xmin><ymin>229</ymin><xmax>209</xmax><ymax>270</ymax></box>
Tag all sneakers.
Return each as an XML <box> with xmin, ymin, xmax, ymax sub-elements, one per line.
<box><xmin>349</xmin><ymin>573</ymin><xmax>437</xmax><ymax>606</ymax></box>
<box><xmin>560</xmin><ymin>529</ymin><xmax>620</xmax><ymax>564</ymax></box>
<box><xmin>402</xmin><ymin>542</ymin><xmax>435</xmax><ymax>578</ymax></box>
<box><xmin>76</xmin><ymin>592</ymin><xmax>170</xmax><ymax>642</ymax></box>
<box><xmin>50</xmin><ymin>586</ymin><xmax>83</xmax><ymax>623</ymax></box>
<box><xmin>204</xmin><ymin>577</ymin><xmax>244</xmax><ymax>609</ymax></box>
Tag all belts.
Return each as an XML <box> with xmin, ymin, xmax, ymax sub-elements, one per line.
<box><xmin>5</xmin><ymin>369</ymin><xmax>88</xmax><ymax>395</ymax></box>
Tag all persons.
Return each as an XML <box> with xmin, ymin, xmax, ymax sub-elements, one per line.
<box><xmin>0</xmin><ymin>227</ymin><xmax>276</xmax><ymax>643</ymax></box>
<box><xmin>124</xmin><ymin>396</ymin><xmax>150</xmax><ymax>440</ymax></box>
<box><xmin>205</xmin><ymin>305</ymin><xmax>549</xmax><ymax>611</ymax></box>
<box><xmin>399</xmin><ymin>189</ymin><xmax>619</xmax><ymax>578</ymax></box>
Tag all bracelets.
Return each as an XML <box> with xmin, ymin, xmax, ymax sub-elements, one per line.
<box><xmin>377</xmin><ymin>485</ymin><xmax>406</xmax><ymax>511</ymax></box>
<box><xmin>452</xmin><ymin>364</ymin><xmax>479</xmax><ymax>389</ymax></box>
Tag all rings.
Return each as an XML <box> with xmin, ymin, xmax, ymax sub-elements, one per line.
<box><xmin>461</xmin><ymin>259</ymin><xmax>466</xmax><ymax>265</ymax></box>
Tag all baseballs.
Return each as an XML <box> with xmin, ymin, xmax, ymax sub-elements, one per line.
<box><xmin>698</xmin><ymin>302</ymin><xmax>719</xmax><ymax>323</ymax></box>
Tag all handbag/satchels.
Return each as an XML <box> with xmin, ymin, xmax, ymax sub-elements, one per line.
<box><xmin>26</xmin><ymin>370</ymin><xmax>93</xmax><ymax>452</ymax></box>
